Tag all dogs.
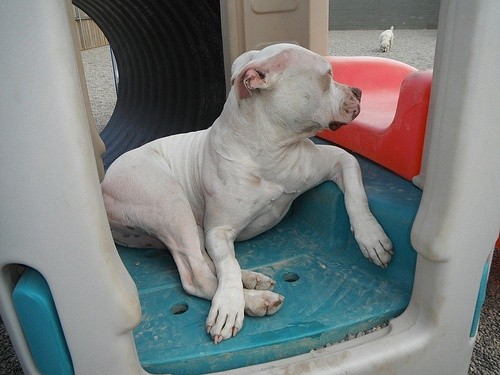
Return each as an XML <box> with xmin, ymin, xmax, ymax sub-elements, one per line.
<box><xmin>378</xmin><ymin>25</ymin><xmax>394</xmax><ymax>53</ymax></box>
<box><xmin>99</xmin><ymin>42</ymin><xmax>395</xmax><ymax>343</ymax></box>
